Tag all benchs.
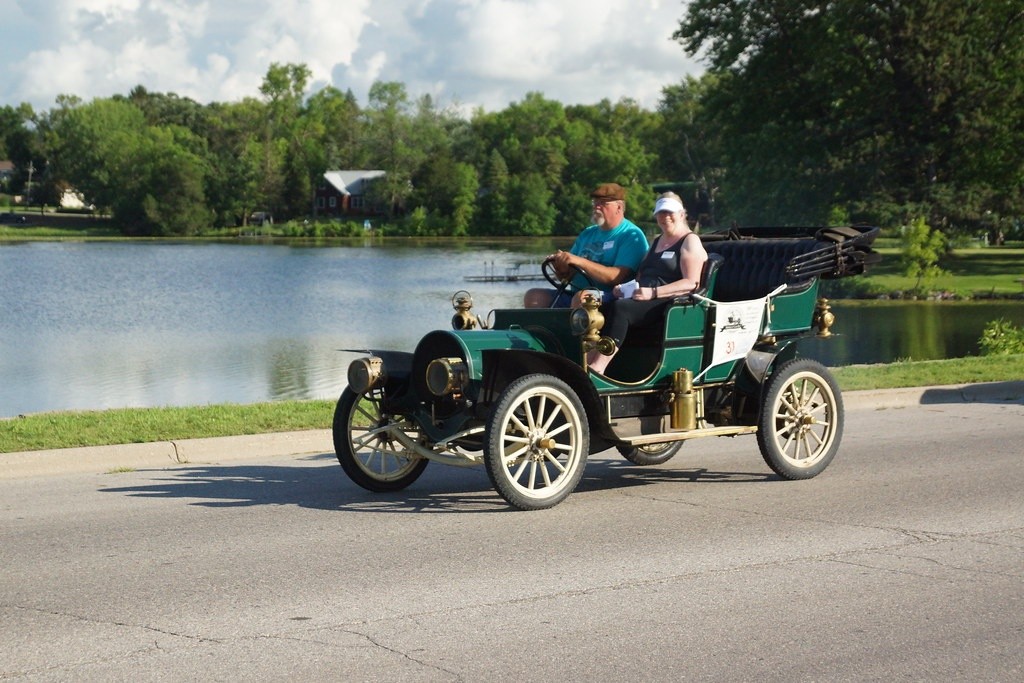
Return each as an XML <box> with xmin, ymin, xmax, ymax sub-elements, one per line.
<box><xmin>701</xmin><ymin>239</ymin><xmax>839</xmax><ymax>336</ymax></box>
<box><xmin>603</xmin><ymin>252</ymin><xmax>725</xmax><ymax>391</ymax></box>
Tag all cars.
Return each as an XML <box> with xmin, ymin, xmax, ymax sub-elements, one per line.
<box><xmin>331</xmin><ymin>223</ymin><xmax>881</xmax><ymax>511</ymax></box>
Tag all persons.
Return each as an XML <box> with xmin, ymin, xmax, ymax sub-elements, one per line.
<box><xmin>523</xmin><ymin>183</ymin><xmax>650</xmax><ymax>308</ymax></box>
<box><xmin>586</xmin><ymin>192</ymin><xmax>713</xmax><ymax>375</ymax></box>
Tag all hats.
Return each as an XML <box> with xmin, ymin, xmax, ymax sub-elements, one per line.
<box><xmin>654</xmin><ymin>198</ymin><xmax>683</xmax><ymax>215</ymax></box>
<box><xmin>590</xmin><ymin>183</ymin><xmax>625</xmax><ymax>200</ymax></box>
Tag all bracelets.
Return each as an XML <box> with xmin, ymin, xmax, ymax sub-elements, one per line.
<box><xmin>653</xmin><ymin>286</ymin><xmax>657</xmax><ymax>299</ymax></box>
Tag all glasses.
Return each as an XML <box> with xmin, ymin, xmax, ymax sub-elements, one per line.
<box><xmin>592</xmin><ymin>201</ymin><xmax>618</xmax><ymax>206</ymax></box>
